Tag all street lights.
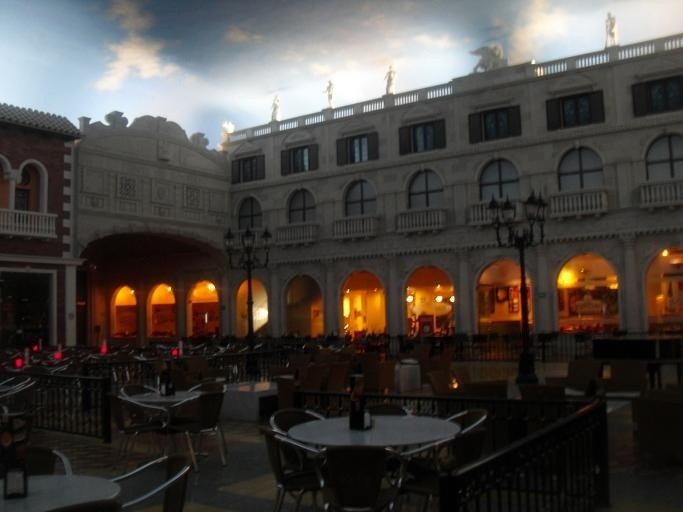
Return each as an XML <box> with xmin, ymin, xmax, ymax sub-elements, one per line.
<box><xmin>222</xmin><ymin>225</ymin><xmax>272</xmax><ymax>352</ymax></box>
<box><xmin>487</xmin><ymin>191</ymin><xmax>554</xmax><ymax>389</ymax></box>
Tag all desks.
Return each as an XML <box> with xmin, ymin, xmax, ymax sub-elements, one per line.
<box><xmin>1</xmin><ymin>472</ymin><xmax>125</xmax><ymax>511</ymax></box>
<box><xmin>286</xmin><ymin>412</ymin><xmax>460</xmax><ymax>511</ymax></box>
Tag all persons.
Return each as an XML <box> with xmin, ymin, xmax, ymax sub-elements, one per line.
<box><xmin>383</xmin><ymin>64</ymin><xmax>395</xmax><ymax>95</ymax></box>
<box><xmin>602</xmin><ymin>12</ymin><xmax>619</xmax><ymax>44</ymax></box>
<box><xmin>322</xmin><ymin>78</ymin><xmax>333</xmax><ymax>105</ymax></box>
<box><xmin>271</xmin><ymin>94</ymin><xmax>280</xmax><ymax>121</ymax></box>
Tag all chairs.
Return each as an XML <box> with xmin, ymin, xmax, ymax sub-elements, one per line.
<box><xmin>1</xmin><ymin>445</ymin><xmax>74</xmax><ymax>476</ymax></box>
<box><xmin>168</xmin><ymin>391</ymin><xmax>228</xmax><ymax>473</ymax></box>
<box><xmin>105</xmin><ymin>428</ymin><xmax>199</xmax><ymax>510</ymax></box>
<box><xmin>1</xmin><ymin>345</ymin><xmax>647</xmax><ymax>447</ymax></box>
<box><xmin>104</xmin><ymin>394</ymin><xmax>168</xmax><ymax>462</ymax></box>
<box><xmin>256</xmin><ymin>423</ymin><xmax>328</xmax><ymax>511</ymax></box>
<box><xmin>388</xmin><ymin>404</ymin><xmax>489</xmax><ymax>508</ymax></box>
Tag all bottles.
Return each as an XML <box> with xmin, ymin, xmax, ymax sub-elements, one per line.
<box><xmin>349</xmin><ymin>374</ymin><xmax>367</xmax><ymax>431</ymax></box>
